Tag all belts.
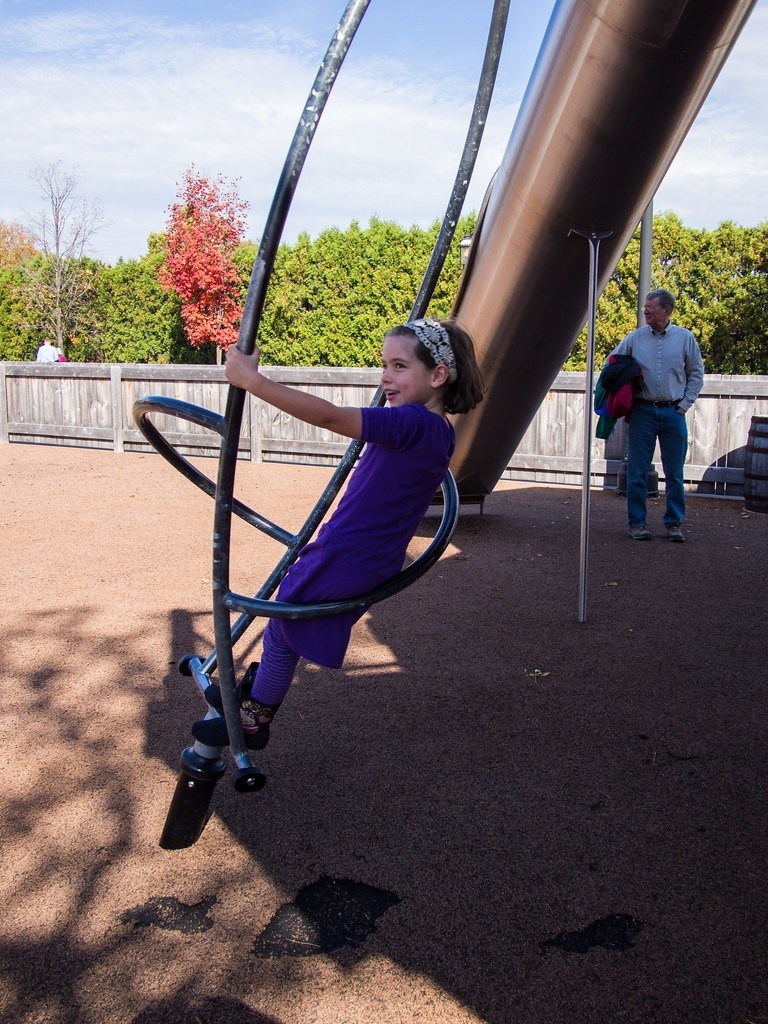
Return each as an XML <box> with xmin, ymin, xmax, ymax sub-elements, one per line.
<box><xmin>635</xmin><ymin>398</ymin><xmax>681</xmax><ymax>407</ymax></box>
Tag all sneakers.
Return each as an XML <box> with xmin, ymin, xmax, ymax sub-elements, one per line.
<box><xmin>665</xmin><ymin>522</ymin><xmax>686</xmax><ymax>541</ymax></box>
<box><xmin>626</xmin><ymin>525</ymin><xmax>655</xmax><ymax>539</ymax></box>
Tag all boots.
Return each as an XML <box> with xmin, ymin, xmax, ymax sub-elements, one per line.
<box><xmin>203</xmin><ymin>661</ymin><xmax>259</xmax><ymax>718</ymax></box>
<box><xmin>191</xmin><ymin>696</ymin><xmax>281</xmax><ymax>750</ymax></box>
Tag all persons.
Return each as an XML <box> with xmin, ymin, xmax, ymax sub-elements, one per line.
<box><xmin>38</xmin><ymin>339</ymin><xmax>58</xmax><ymax>362</ymax></box>
<box><xmin>190</xmin><ymin>319</ymin><xmax>482</xmax><ymax>750</ymax></box>
<box><xmin>604</xmin><ymin>288</ymin><xmax>704</xmax><ymax>543</ymax></box>
